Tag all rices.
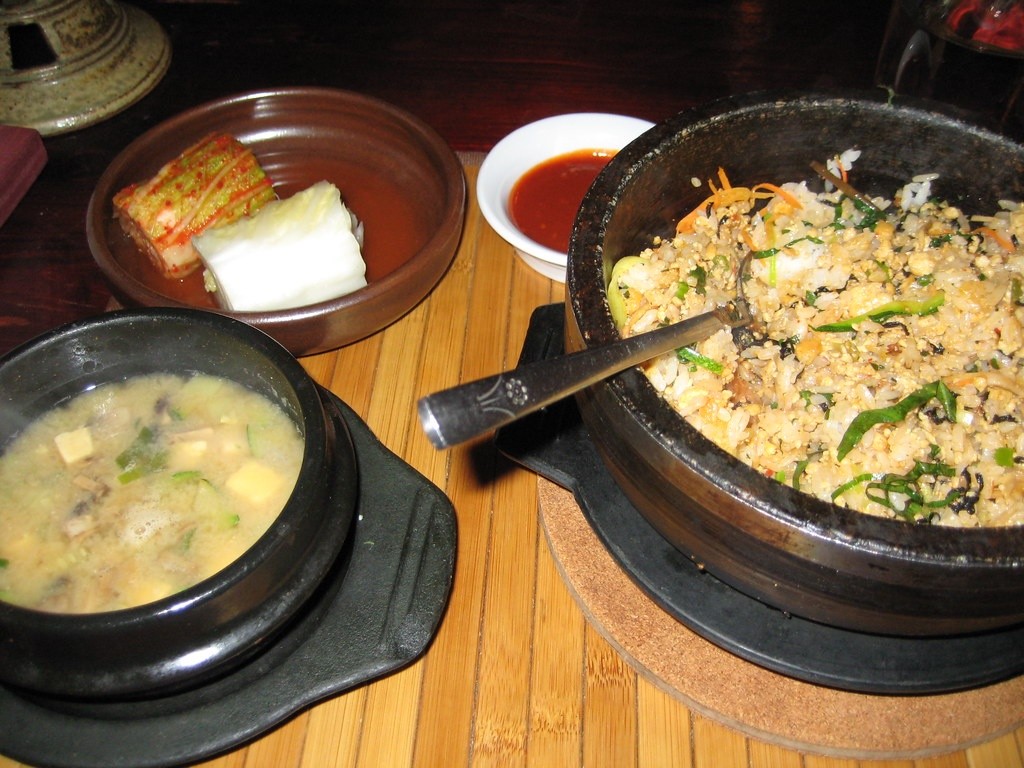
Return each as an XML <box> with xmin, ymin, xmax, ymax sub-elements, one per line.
<box><xmin>608</xmin><ymin>149</ymin><xmax>1024</xmax><ymax>527</ymax></box>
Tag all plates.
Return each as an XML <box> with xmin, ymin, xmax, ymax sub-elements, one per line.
<box><xmin>1</xmin><ymin>388</ymin><xmax>458</xmax><ymax>768</ymax></box>
<box><xmin>497</xmin><ymin>301</ymin><xmax>1024</xmax><ymax>694</ymax></box>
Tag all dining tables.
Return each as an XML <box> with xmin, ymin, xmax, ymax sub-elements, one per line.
<box><xmin>0</xmin><ymin>152</ymin><xmax>1024</xmax><ymax>768</ymax></box>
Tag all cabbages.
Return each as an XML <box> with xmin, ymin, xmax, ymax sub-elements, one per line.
<box><xmin>109</xmin><ymin>133</ymin><xmax>369</xmax><ymax>313</ymax></box>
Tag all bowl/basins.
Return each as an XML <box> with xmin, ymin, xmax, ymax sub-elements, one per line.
<box><xmin>84</xmin><ymin>87</ymin><xmax>466</xmax><ymax>357</ymax></box>
<box><xmin>565</xmin><ymin>92</ymin><xmax>1024</xmax><ymax>635</ymax></box>
<box><xmin>1</xmin><ymin>306</ymin><xmax>361</xmax><ymax>716</ymax></box>
<box><xmin>474</xmin><ymin>112</ymin><xmax>657</xmax><ymax>286</ymax></box>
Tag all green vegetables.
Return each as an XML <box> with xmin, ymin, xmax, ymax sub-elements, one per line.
<box><xmin>665</xmin><ymin>191</ymin><xmax>1021</xmax><ymax>524</ymax></box>
<box><xmin>115</xmin><ymin>411</ymin><xmax>240</xmax><ymax>549</ymax></box>
